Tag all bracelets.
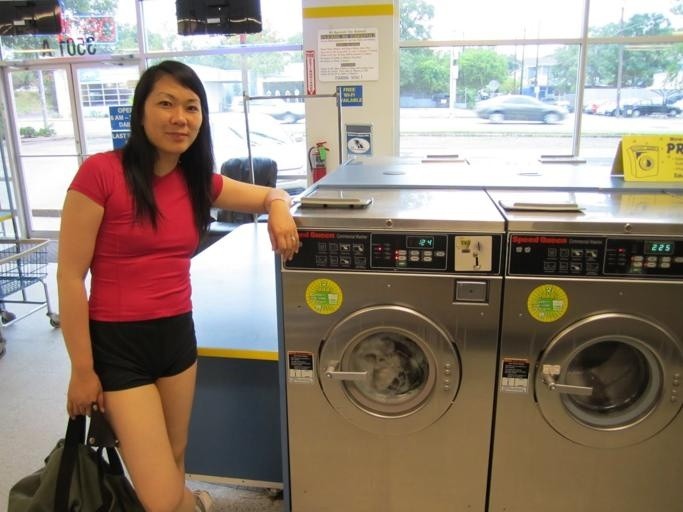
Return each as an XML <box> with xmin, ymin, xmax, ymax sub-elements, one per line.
<box><xmin>270</xmin><ymin>198</ymin><xmax>286</xmax><ymax>203</ymax></box>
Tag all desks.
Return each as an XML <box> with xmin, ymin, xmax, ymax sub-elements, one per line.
<box><xmin>190</xmin><ymin>222</ymin><xmax>279</xmax><ymax>360</ymax></box>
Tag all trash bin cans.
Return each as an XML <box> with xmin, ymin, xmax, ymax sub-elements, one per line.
<box><xmin>435</xmin><ymin>93</ymin><xmax>449</xmax><ymax>107</ymax></box>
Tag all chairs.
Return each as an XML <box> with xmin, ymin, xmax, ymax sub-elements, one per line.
<box><xmin>218</xmin><ymin>157</ymin><xmax>278</xmax><ymax>222</ymax></box>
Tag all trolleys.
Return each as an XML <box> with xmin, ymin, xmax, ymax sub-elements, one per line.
<box><xmin>0</xmin><ymin>234</ymin><xmax>58</xmax><ymax>359</ymax></box>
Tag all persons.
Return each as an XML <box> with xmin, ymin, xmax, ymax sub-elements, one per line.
<box><xmin>56</xmin><ymin>60</ymin><xmax>300</xmax><ymax>512</ymax></box>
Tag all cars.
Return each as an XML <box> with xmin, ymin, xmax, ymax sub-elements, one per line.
<box><xmin>540</xmin><ymin>79</ymin><xmax>681</xmax><ymax>123</ymax></box>
<box><xmin>473</xmin><ymin>94</ymin><xmax>566</xmax><ymax>124</ymax></box>
<box><xmin>236</xmin><ymin>97</ymin><xmax>305</xmax><ymax>123</ymax></box>
<box><xmin>208</xmin><ymin>109</ymin><xmax>307</xmax><ymax>181</ymax></box>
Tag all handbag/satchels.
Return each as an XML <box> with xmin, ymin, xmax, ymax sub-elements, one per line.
<box><xmin>9</xmin><ymin>438</ymin><xmax>146</xmax><ymax>512</ymax></box>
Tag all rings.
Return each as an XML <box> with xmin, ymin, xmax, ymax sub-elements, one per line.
<box><xmin>291</xmin><ymin>237</ymin><xmax>297</xmax><ymax>242</ymax></box>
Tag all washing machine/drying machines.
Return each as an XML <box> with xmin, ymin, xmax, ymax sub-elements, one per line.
<box><xmin>277</xmin><ymin>152</ymin><xmax>501</xmax><ymax>511</ymax></box>
<box><xmin>503</xmin><ymin>153</ymin><xmax>683</xmax><ymax>512</ymax></box>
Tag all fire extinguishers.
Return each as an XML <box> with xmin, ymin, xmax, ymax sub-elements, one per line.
<box><xmin>308</xmin><ymin>141</ymin><xmax>330</xmax><ymax>183</ymax></box>
